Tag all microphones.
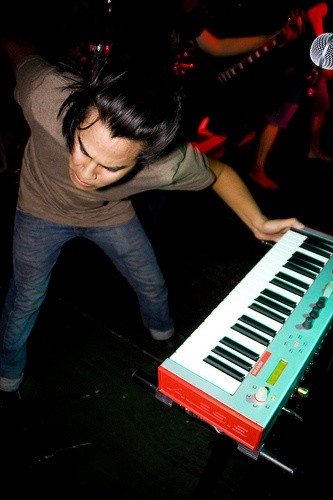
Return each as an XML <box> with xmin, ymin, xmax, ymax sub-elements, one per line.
<box><xmin>310</xmin><ymin>33</ymin><xmax>333</xmax><ymax>70</ymax></box>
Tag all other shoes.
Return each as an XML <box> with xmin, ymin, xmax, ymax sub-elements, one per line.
<box><xmin>0</xmin><ymin>389</ymin><xmax>21</xmax><ymax>415</ymax></box>
<box><xmin>151</xmin><ymin>333</ymin><xmax>187</xmax><ymax>359</ymax></box>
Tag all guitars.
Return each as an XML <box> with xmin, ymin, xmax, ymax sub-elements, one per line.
<box><xmin>182</xmin><ymin>16</ymin><xmax>303</xmax><ymax>126</ymax></box>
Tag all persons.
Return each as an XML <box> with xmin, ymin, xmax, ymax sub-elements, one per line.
<box><xmin>0</xmin><ymin>27</ymin><xmax>305</xmax><ymax>419</ymax></box>
<box><xmin>95</xmin><ymin>0</ymin><xmax>333</xmax><ymax>201</ymax></box>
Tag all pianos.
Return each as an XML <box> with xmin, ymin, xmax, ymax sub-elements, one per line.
<box><xmin>156</xmin><ymin>227</ymin><xmax>333</xmax><ymax>455</ymax></box>
